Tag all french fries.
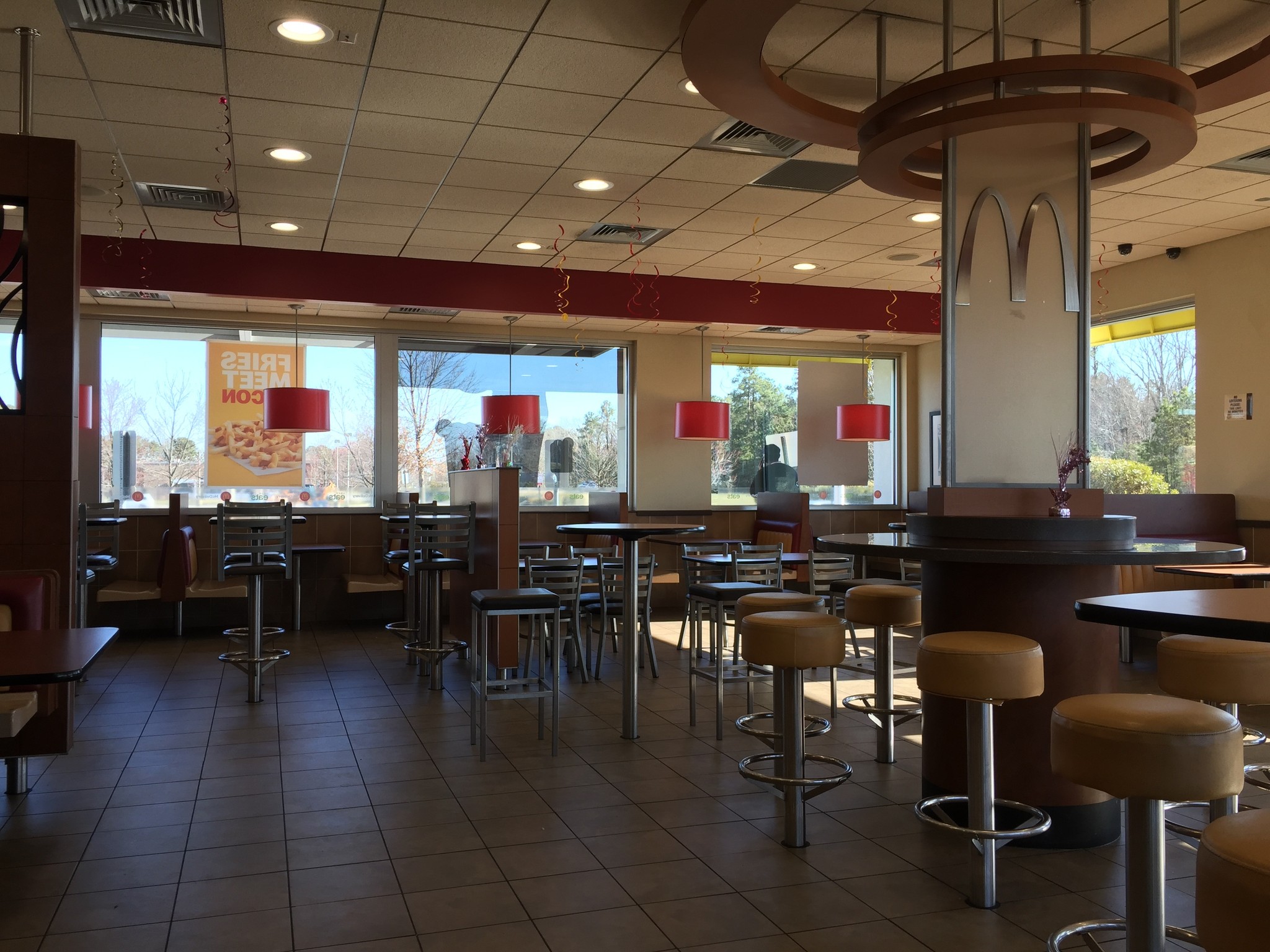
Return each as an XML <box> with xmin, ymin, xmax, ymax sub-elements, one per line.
<box><xmin>207</xmin><ymin>413</ymin><xmax>302</xmax><ymax>469</ymax></box>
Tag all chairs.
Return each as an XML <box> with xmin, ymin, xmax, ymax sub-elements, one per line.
<box><xmin>0</xmin><ymin>568</ymin><xmax>61</xmax><ymax>738</ymax></box>
<box><xmin>95</xmin><ymin>530</ymin><xmax>250</xmax><ymax>603</ymax></box>
<box><xmin>346</xmin><ymin>524</ymin><xmax>408</xmax><ymax>593</ymax></box>
<box><xmin>520</xmin><ymin>521</ymin><xmax>921</xmax><ymax>683</ymax></box>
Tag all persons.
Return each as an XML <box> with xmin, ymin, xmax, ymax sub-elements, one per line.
<box><xmin>326</xmin><ymin>479</ymin><xmax>336</xmax><ymax>488</ymax></box>
<box><xmin>749</xmin><ymin>444</ymin><xmax>801</xmax><ymax>498</ymax></box>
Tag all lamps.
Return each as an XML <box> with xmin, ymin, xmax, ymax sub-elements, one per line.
<box><xmin>674</xmin><ymin>327</ymin><xmax>729</xmax><ymax>439</ymax></box>
<box><xmin>837</xmin><ymin>335</ymin><xmax>891</xmax><ymax>441</ymax></box>
<box><xmin>263</xmin><ymin>305</ymin><xmax>331</xmax><ymax>434</ymax></box>
<box><xmin>481</xmin><ymin>315</ymin><xmax>541</xmax><ymax>436</ymax></box>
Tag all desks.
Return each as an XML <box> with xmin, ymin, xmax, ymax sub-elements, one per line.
<box><xmin>814</xmin><ymin>521</ymin><xmax>1270</xmax><ymax>701</ymax></box>
<box><xmin>78</xmin><ymin>517</ymin><xmax>128</xmax><ymax>537</ymax></box>
<box><xmin>208</xmin><ymin>515</ymin><xmax>314</xmax><ymax>632</ymax></box>
<box><xmin>0</xmin><ymin>627</ymin><xmax>120</xmax><ymax>687</ymax></box>
<box><xmin>278</xmin><ymin>542</ymin><xmax>347</xmax><ymax>632</ymax></box>
<box><xmin>519</xmin><ymin>523</ymin><xmax>751</xmax><ymax>740</ymax></box>
<box><xmin>380</xmin><ymin>514</ymin><xmax>469</xmax><ymax>677</ymax></box>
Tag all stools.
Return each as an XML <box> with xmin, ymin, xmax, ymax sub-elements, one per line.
<box><xmin>217</xmin><ymin>499</ymin><xmax>292</xmax><ymax>702</ymax></box>
<box><xmin>688</xmin><ymin>578</ymin><xmax>1270</xmax><ymax>952</ymax></box>
<box><xmin>382</xmin><ymin>500</ymin><xmax>562</xmax><ymax>762</ymax></box>
<box><xmin>78</xmin><ymin>500</ymin><xmax>120</xmax><ymax>626</ymax></box>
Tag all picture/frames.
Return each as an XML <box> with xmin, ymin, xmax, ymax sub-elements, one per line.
<box><xmin>929</xmin><ymin>411</ymin><xmax>943</xmax><ymax>488</ymax></box>
